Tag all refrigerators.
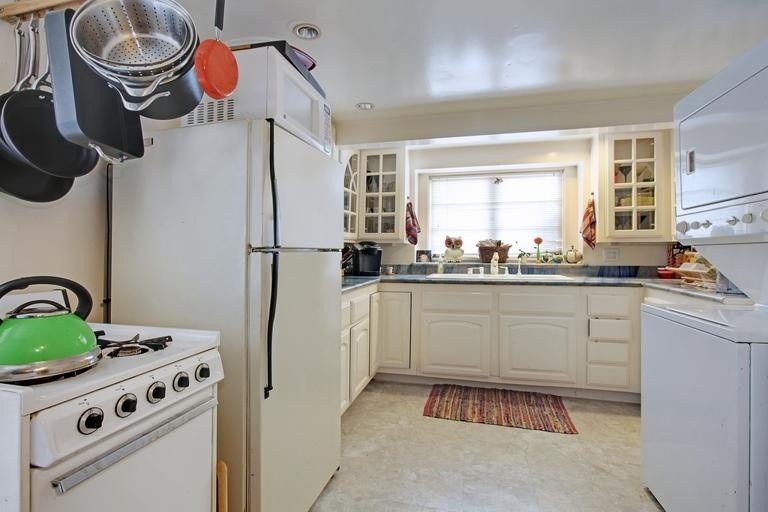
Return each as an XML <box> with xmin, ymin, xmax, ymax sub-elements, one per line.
<box><xmin>105</xmin><ymin>122</ymin><xmax>345</xmax><ymax>512</ymax></box>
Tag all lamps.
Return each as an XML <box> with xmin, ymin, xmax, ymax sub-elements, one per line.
<box><xmin>292</xmin><ymin>23</ymin><xmax>321</xmax><ymax>40</ymax></box>
<box><xmin>353</xmin><ymin>102</ymin><xmax>376</xmax><ymax>113</ymax></box>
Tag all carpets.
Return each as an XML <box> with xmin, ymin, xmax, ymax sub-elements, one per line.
<box><xmin>422</xmin><ymin>384</ymin><xmax>579</xmax><ymax>435</ymax></box>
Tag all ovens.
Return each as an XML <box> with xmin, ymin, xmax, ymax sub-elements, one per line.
<box><xmin>672</xmin><ymin>34</ymin><xmax>768</xmax><ymax>245</ymax></box>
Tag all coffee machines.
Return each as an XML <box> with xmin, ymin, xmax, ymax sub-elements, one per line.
<box><xmin>351</xmin><ymin>241</ymin><xmax>383</xmax><ymax>277</ymax></box>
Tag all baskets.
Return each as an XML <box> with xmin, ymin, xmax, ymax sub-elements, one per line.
<box><xmin>478</xmin><ymin>240</ymin><xmax>512</xmax><ymax>263</ymax></box>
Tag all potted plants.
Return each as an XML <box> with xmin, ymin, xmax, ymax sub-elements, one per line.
<box><xmin>518</xmin><ymin>249</ymin><xmax>531</xmax><ymax>263</ymax></box>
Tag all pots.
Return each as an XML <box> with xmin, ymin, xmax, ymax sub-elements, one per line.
<box><xmin>0</xmin><ymin>7</ymin><xmax>99</xmax><ymax>178</ymax></box>
<box><xmin>68</xmin><ymin>0</ymin><xmax>203</xmax><ymax>122</ymax></box>
<box><xmin>0</xmin><ymin>15</ymin><xmax>76</xmax><ymax>204</ymax></box>
<box><xmin>193</xmin><ymin>0</ymin><xmax>238</xmax><ymax>100</ymax></box>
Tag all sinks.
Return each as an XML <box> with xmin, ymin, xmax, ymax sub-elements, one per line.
<box><xmin>425</xmin><ymin>272</ymin><xmax>572</xmax><ymax>282</ymax></box>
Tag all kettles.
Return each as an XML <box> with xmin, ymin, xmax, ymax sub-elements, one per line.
<box><xmin>0</xmin><ymin>275</ymin><xmax>100</xmax><ymax>386</ymax></box>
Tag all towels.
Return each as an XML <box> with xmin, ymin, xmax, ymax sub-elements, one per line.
<box><xmin>580</xmin><ymin>199</ymin><xmax>596</xmax><ymax>250</ymax></box>
<box><xmin>405</xmin><ymin>204</ymin><xmax>421</xmax><ymax>246</ymax></box>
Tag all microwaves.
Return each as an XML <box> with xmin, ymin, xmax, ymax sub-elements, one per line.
<box><xmin>177</xmin><ymin>45</ymin><xmax>331</xmax><ymax>156</ymax></box>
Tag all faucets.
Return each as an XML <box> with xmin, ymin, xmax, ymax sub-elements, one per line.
<box><xmin>490</xmin><ymin>252</ymin><xmax>500</xmax><ymax>274</ymax></box>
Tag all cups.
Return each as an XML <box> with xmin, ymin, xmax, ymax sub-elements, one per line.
<box><xmin>630</xmin><ymin>214</ymin><xmax>649</xmax><ymax>229</ymax></box>
<box><xmin>619</xmin><ymin>166</ymin><xmax>632</xmax><ymax>183</ymax></box>
<box><xmin>616</xmin><ymin>214</ymin><xmax>631</xmax><ymax>230</ymax></box>
<box><xmin>636</xmin><ymin>165</ymin><xmax>647</xmax><ymax>182</ymax></box>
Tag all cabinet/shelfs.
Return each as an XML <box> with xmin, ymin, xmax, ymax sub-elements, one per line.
<box><xmin>586</xmin><ymin>291</ymin><xmax>631</xmax><ymax>388</ymax></box>
<box><xmin>370</xmin><ymin>291</ymin><xmax>380</xmax><ymax>378</ymax></box>
<box><xmin>590</xmin><ymin>130</ymin><xmax>672</xmax><ymax>246</ymax></box>
<box><xmin>377</xmin><ymin>291</ymin><xmax>412</xmax><ymax>369</ymax></box>
<box><xmin>360</xmin><ymin>148</ymin><xmax>410</xmax><ymax>245</ymax></box>
<box><xmin>344</xmin><ymin>151</ymin><xmax>358</xmax><ymax>240</ymax></box>
<box><xmin>419</xmin><ymin>291</ymin><xmax>493</xmax><ymax>379</ymax></box>
<box><xmin>341</xmin><ymin>294</ymin><xmax>370</xmax><ymax>416</ymax></box>
<box><xmin>498</xmin><ymin>291</ymin><xmax>577</xmax><ymax>385</ymax></box>
